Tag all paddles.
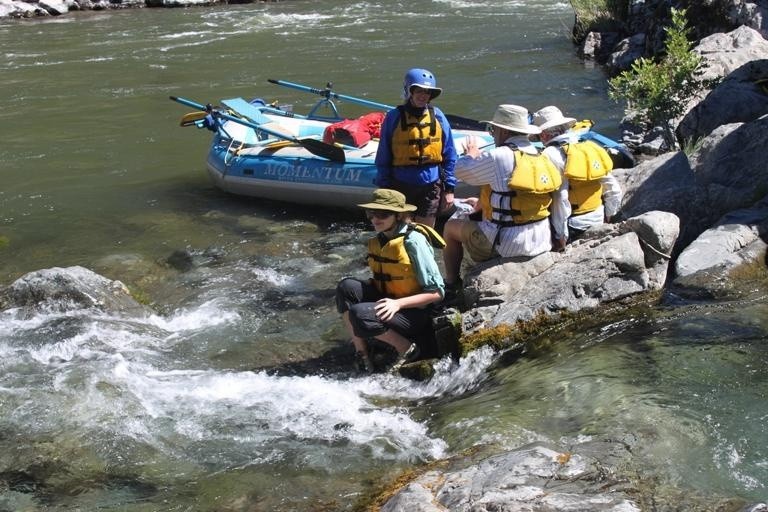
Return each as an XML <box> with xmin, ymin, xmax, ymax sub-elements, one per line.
<box><xmin>169</xmin><ymin>96</ymin><xmax>345</xmax><ymax>165</ymax></box>
<box><xmin>266</xmin><ymin>79</ymin><xmax>481</xmax><ymax>131</ymax></box>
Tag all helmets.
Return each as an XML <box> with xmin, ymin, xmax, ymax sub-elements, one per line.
<box><xmin>403</xmin><ymin>68</ymin><xmax>443</xmax><ymax>100</ymax></box>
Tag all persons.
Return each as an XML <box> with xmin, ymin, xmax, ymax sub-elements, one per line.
<box><xmin>442</xmin><ymin>102</ymin><xmax>563</xmax><ymax>287</ymax></box>
<box><xmin>333</xmin><ymin>187</ymin><xmax>446</xmax><ymax>373</ymax></box>
<box><xmin>526</xmin><ymin>104</ymin><xmax>622</xmax><ymax>255</ymax></box>
<box><xmin>376</xmin><ymin>67</ymin><xmax>458</xmax><ymax>230</ymax></box>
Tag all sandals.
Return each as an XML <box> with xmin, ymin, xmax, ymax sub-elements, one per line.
<box><xmin>383</xmin><ymin>342</ymin><xmax>421</xmax><ymax>372</ymax></box>
<box><xmin>349</xmin><ymin>350</ymin><xmax>375</xmax><ymax>381</ymax></box>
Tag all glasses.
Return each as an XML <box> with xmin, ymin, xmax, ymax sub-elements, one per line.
<box><xmin>414</xmin><ymin>88</ymin><xmax>432</xmax><ymax>94</ymax></box>
<box><xmin>487</xmin><ymin>125</ymin><xmax>494</xmax><ymax>136</ymax></box>
<box><xmin>365</xmin><ymin>209</ymin><xmax>392</xmax><ymax>220</ymax></box>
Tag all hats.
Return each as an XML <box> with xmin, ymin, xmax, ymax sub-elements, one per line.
<box><xmin>479</xmin><ymin>104</ymin><xmax>542</xmax><ymax>135</ymax></box>
<box><xmin>357</xmin><ymin>189</ymin><xmax>417</xmax><ymax>213</ymax></box>
<box><xmin>528</xmin><ymin>105</ymin><xmax>577</xmax><ymax>142</ymax></box>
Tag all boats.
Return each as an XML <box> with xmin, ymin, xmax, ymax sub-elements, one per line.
<box><xmin>204</xmin><ymin>97</ymin><xmax>635</xmax><ymax>208</ymax></box>
